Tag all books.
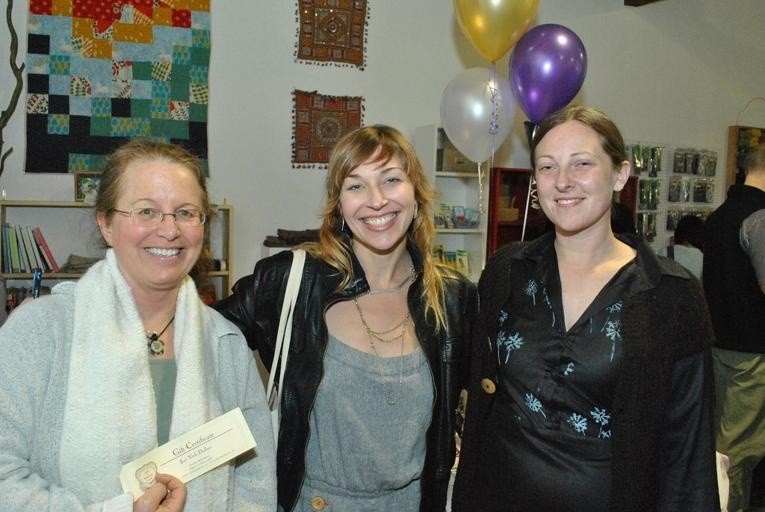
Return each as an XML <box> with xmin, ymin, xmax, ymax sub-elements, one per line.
<box><xmin>2</xmin><ymin>225</ymin><xmax>100</xmax><ymax>315</ymax></box>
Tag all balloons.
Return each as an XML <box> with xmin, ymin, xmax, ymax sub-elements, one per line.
<box><xmin>508</xmin><ymin>24</ymin><xmax>587</xmax><ymax>125</ymax></box>
<box><xmin>452</xmin><ymin>0</ymin><xmax>542</xmax><ymax>64</ymax></box>
<box><xmin>441</xmin><ymin>69</ymin><xmax>517</xmax><ymax>166</ymax></box>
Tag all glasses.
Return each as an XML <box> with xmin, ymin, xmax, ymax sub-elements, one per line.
<box><xmin>113</xmin><ymin>207</ymin><xmax>206</xmax><ymax>228</ymax></box>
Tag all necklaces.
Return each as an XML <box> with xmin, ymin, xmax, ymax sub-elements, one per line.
<box><xmin>147</xmin><ymin>315</ymin><xmax>174</xmax><ymax>356</ymax></box>
<box><xmin>353</xmin><ymin>264</ymin><xmax>419</xmax><ymax>406</ymax></box>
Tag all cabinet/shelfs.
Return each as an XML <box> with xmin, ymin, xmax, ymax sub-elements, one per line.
<box><xmin>415</xmin><ymin>124</ymin><xmax>492</xmax><ymax>283</ymax></box>
<box><xmin>1</xmin><ymin>199</ymin><xmax>235</xmax><ymax>326</ymax></box>
<box><xmin>486</xmin><ymin>166</ymin><xmax>640</xmax><ymax>271</ymax></box>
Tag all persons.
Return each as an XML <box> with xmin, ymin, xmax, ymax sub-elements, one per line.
<box><xmin>704</xmin><ymin>143</ymin><xmax>765</xmax><ymax>512</ymax></box>
<box><xmin>655</xmin><ymin>215</ymin><xmax>704</xmax><ymax>289</ymax></box>
<box><xmin>0</xmin><ymin>140</ymin><xmax>279</xmax><ymax>511</ymax></box>
<box><xmin>208</xmin><ymin>125</ymin><xmax>475</xmax><ymax>512</ymax></box>
<box><xmin>462</xmin><ymin>107</ymin><xmax>716</xmax><ymax>509</ymax></box>
